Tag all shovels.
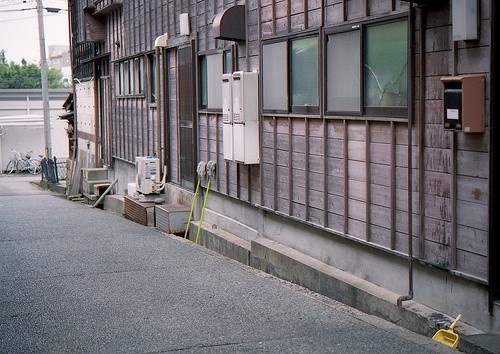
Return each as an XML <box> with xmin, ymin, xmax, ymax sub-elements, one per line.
<box><xmin>430</xmin><ymin>313</ymin><xmax>463</xmax><ymax>348</ymax></box>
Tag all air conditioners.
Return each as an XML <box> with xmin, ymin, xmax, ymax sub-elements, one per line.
<box><xmin>132</xmin><ymin>153</ymin><xmax>163</xmax><ymax>195</ymax></box>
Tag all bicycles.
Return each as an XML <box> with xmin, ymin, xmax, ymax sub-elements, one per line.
<box><xmin>16</xmin><ymin>150</ymin><xmax>44</xmax><ymax>174</ymax></box>
<box><xmin>6</xmin><ymin>148</ymin><xmax>42</xmax><ymax>175</ymax></box>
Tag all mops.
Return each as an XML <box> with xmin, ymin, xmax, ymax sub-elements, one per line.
<box><xmin>184</xmin><ymin>160</ymin><xmax>216</xmax><ymax>244</ymax></box>
